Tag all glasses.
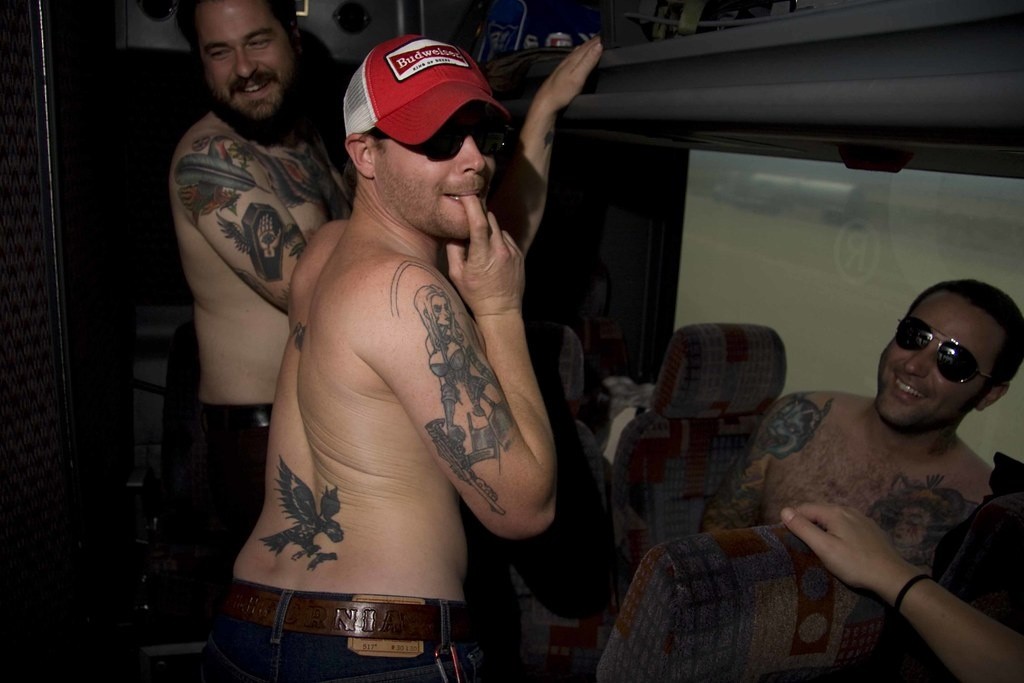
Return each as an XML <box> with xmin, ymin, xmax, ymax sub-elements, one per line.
<box><xmin>368</xmin><ymin>128</ymin><xmax>504</xmax><ymax>161</ymax></box>
<box><xmin>896</xmin><ymin>317</ymin><xmax>990</xmax><ymax>384</ymax></box>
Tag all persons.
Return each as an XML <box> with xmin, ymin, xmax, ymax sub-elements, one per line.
<box><xmin>780</xmin><ymin>501</ymin><xmax>1024</xmax><ymax>683</ymax></box>
<box><xmin>168</xmin><ymin>0</ymin><xmax>604</xmax><ymax>683</ymax></box>
<box><xmin>701</xmin><ymin>278</ymin><xmax>1024</xmax><ymax>577</ymax></box>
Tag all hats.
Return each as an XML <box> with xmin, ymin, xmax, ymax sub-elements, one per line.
<box><xmin>343</xmin><ymin>34</ymin><xmax>508</xmax><ymax>144</ymax></box>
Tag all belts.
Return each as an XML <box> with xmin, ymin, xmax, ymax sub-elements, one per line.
<box><xmin>202</xmin><ymin>404</ymin><xmax>267</xmax><ymax>428</ymax></box>
<box><xmin>225</xmin><ymin>584</ymin><xmax>471</xmax><ymax>645</ymax></box>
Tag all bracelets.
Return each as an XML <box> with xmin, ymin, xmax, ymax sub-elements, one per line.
<box><xmin>894</xmin><ymin>574</ymin><xmax>935</xmax><ymax>611</ymax></box>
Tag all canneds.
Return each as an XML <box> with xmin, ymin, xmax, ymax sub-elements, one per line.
<box><xmin>545</xmin><ymin>32</ymin><xmax>573</xmax><ymax>47</ymax></box>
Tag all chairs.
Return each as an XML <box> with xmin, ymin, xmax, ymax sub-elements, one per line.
<box><xmin>485</xmin><ymin>324</ymin><xmax>1024</xmax><ymax>683</ymax></box>
